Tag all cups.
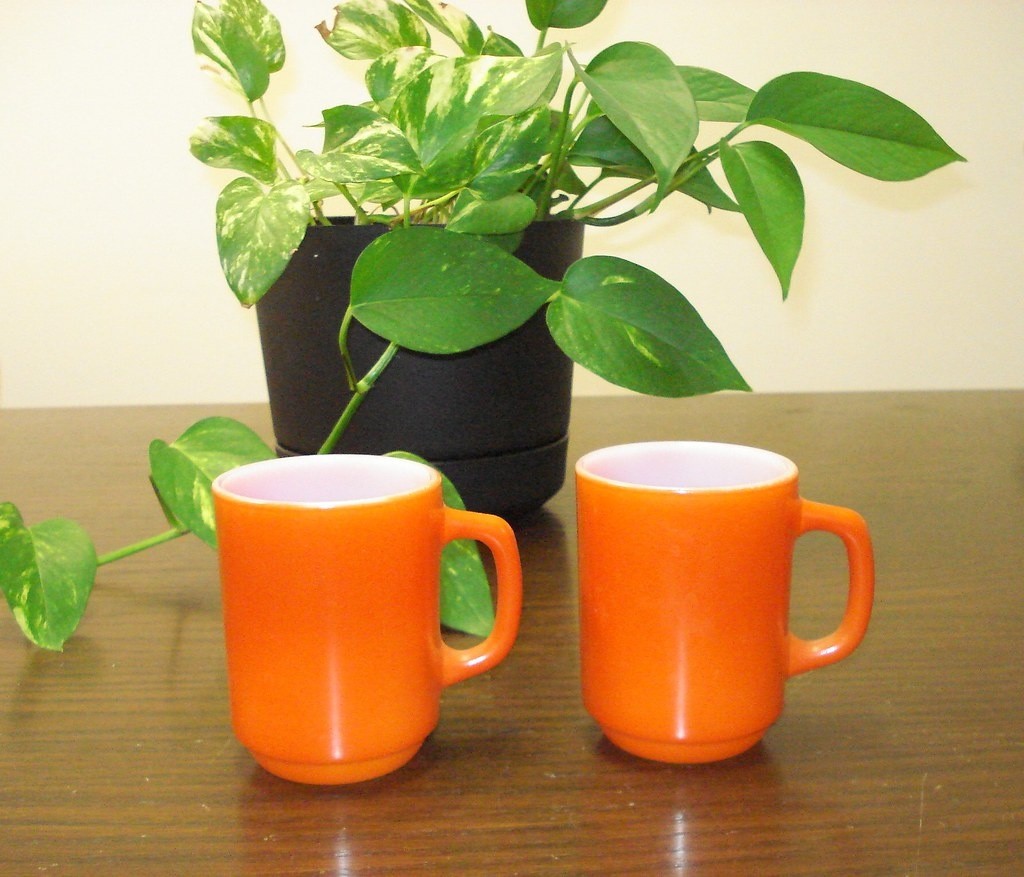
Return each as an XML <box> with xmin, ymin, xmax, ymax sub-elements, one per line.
<box><xmin>208</xmin><ymin>454</ymin><xmax>524</xmax><ymax>786</ymax></box>
<box><xmin>572</xmin><ymin>442</ymin><xmax>874</xmax><ymax>764</ymax></box>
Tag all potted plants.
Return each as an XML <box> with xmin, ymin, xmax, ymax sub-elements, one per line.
<box><xmin>0</xmin><ymin>0</ymin><xmax>966</xmax><ymax>654</ymax></box>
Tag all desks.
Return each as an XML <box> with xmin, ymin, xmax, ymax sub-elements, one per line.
<box><xmin>0</xmin><ymin>382</ymin><xmax>1024</xmax><ymax>877</ymax></box>
